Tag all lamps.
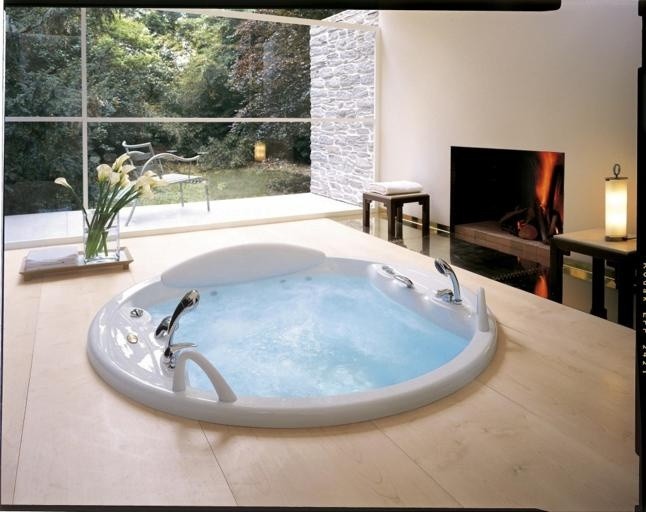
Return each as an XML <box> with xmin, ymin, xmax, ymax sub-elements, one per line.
<box><xmin>604</xmin><ymin>164</ymin><xmax>628</xmax><ymax>242</ymax></box>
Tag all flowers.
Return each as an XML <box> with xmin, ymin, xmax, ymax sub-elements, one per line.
<box><xmin>54</xmin><ymin>152</ymin><xmax>169</xmax><ymax>259</ymax></box>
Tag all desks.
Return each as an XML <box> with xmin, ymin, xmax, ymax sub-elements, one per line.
<box><xmin>362</xmin><ymin>191</ymin><xmax>430</xmax><ymax>251</ymax></box>
<box><xmin>550</xmin><ymin>227</ymin><xmax>637</xmax><ymax>328</ymax></box>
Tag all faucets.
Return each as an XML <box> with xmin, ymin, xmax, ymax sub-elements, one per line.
<box><xmin>433</xmin><ymin>255</ymin><xmax>463</xmax><ymax>303</ymax></box>
<box><xmin>161</xmin><ymin>285</ymin><xmax>200</xmax><ymax>372</ymax></box>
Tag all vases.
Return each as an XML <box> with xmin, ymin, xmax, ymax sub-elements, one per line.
<box><xmin>82</xmin><ymin>210</ymin><xmax>120</xmax><ymax>264</ymax></box>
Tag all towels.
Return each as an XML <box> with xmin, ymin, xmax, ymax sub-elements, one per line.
<box><xmin>365</xmin><ymin>181</ymin><xmax>423</xmax><ymax>196</ymax></box>
<box><xmin>22</xmin><ymin>246</ymin><xmax>85</xmax><ymax>270</ymax></box>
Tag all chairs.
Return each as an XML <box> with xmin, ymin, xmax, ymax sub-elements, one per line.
<box><xmin>122</xmin><ymin>139</ymin><xmax>210</xmax><ymax>226</ymax></box>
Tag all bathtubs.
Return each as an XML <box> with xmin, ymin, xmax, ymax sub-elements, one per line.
<box><xmin>85</xmin><ymin>242</ymin><xmax>498</xmax><ymax>432</ymax></box>
